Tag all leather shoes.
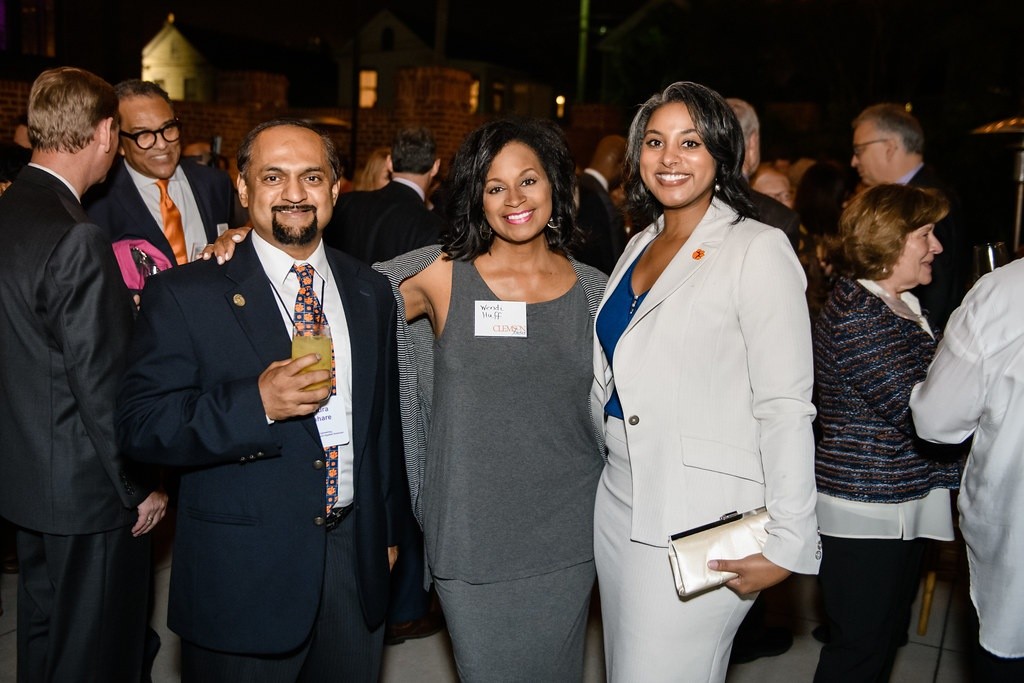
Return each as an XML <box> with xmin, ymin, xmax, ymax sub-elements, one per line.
<box><xmin>387</xmin><ymin>599</ymin><xmax>446</xmax><ymax>648</ymax></box>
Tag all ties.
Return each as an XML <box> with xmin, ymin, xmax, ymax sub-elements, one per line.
<box><xmin>155</xmin><ymin>179</ymin><xmax>188</xmax><ymax>266</ymax></box>
<box><xmin>292</xmin><ymin>263</ymin><xmax>339</xmax><ymax>517</ymax></box>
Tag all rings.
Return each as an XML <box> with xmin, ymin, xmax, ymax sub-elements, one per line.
<box><xmin>206</xmin><ymin>243</ymin><xmax>214</xmax><ymax>247</ymax></box>
<box><xmin>148</xmin><ymin>517</ymin><xmax>153</xmax><ymax>521</ymax></box>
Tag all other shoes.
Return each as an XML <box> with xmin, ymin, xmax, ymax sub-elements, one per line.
<box><xmin>730</xmin><ymin>621</ymin><xmax>796</xmax><ymax>664</ymax></box>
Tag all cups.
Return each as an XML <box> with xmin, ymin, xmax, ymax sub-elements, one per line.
<box><xmin>973</xmin><ymin>240</ymin><xmax>1009</xmax><ymax>279</ymax></box>
<box><xmin>190</xmin><ymin>242</ymin><xmax>209</xmax><ymax>262</ymax></box>
<box><xmin>291</xmin><ymin>323</ymin><xmax>332</xmax><ymax>403</ymax></box>
<box><xmin>142</xmin><ymin>262</ymin><xmax>168</xmax><ymax>279</ymax></box>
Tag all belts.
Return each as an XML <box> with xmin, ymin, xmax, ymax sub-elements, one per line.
<box><xmin>325</xmin><ymin>502</ymin><xmax>354</xmax><ymax>531</ymax></box>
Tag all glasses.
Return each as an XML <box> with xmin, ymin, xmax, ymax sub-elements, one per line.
<box><xmin>118</xmin><ymin>118</ymin><xmax>182</xmax><ymax>150</ymax></box>
<box><xmin>853</xmin><ymin>138</ymin><xmax>898</xmax><ymax>158</ymax></box>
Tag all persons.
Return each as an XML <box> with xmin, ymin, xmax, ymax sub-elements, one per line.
<box><xmin>810</xmin><ymin>182</ymin><xmax>962</xmax><ymax>683</ymax></box>
<box><xmin>590</xmin><ymin>83</ymin><xmax>824</xmax><ymax>683</ymax></box>
<box><xmin>908</xmin><ymin>256</ymin><xmax>1024</xmax><ymax>683</ymax></box>
<box><xmin>321</xmin><ymin>128</ymin><xmax>630</xmax><ymax>277</ymax></box>
<box><xmin>194</xmin><ymin>116</ymin><xmax>610</xmax><ymax>683</ymax></box>
<box><xmin>80</xmin><ymin>80</ymin><xmax>238</xmax><ymax>316</ymax></box>
<box><xmin>724</xmin><ymin>98</ymin><xmax>976</xmax><ymax>329</ymax></box>
<box><xmin>0</xmin><ymin>65</ymin><xmax>168</xmax><ymax>683</ymax></box>
<box><xmin>114</xmin><ymin>116</ymin><xmax>399</xmax><ymax>683</ymax></box>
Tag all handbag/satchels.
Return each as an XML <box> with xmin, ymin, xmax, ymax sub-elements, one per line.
<box><xmin>667</xmin><ymin>505</ymin><xmax>774</xmax><ymax>597</ymax></box>
